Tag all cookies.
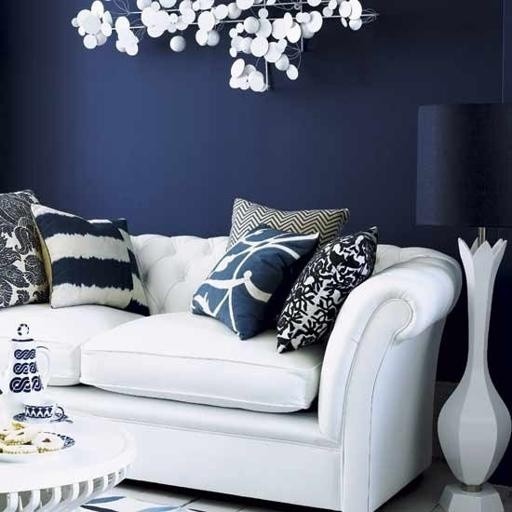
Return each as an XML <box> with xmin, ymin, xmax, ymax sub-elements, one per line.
<box><xmin>0</xmin><ymin>426</ymin><xmax>65</xmax><ymax>455</ymax></box>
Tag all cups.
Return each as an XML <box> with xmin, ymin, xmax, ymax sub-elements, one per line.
<box><xmin>24</xmin><ymin>399</ymin><xmax>65</xmax><ymax>424</ymax></box>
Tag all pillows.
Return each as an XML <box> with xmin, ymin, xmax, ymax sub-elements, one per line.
<box><xmin>187</xmin><ymin>197</ymin><xmax>380</xmax><ymax>354</ymax></box>
<box><xmin>0</xmin><ymin>187</ymin><xmax>154</xmax><ymax>318</ymax></box>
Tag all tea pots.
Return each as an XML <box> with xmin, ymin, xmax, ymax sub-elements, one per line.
<box><xmin>0</xmin><ymin>323</ymin><xmax>53</xmax><ymax>404</ymax></box>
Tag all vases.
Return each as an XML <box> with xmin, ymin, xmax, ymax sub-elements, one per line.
<box><xmin>432</xmin><ymin>235</ymin><xmax>512</xmax><ymax>511</ymax></box>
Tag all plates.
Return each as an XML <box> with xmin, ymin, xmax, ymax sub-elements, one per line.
<box><xmin>0</xmin><ymin>431</ymin><xmax>77</xmax><ymax>454</ymax></box>
<box><xmin>12</xmin><ymin>410</ymin><xmax>77</xmax><ymax>428</ymax></box>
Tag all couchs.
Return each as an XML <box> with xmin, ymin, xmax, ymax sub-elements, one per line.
<box><xmin>2</xmin><ymin>234</ymin><xmax>465</xmax><ymax>512</ymax></box>
